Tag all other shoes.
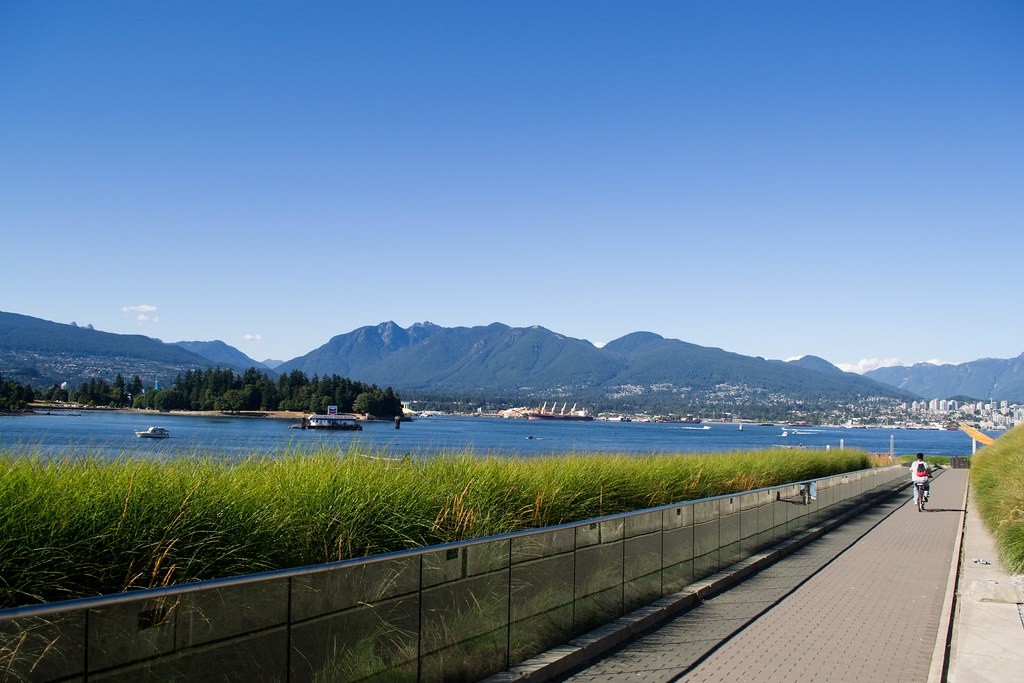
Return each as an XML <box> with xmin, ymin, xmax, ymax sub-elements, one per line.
<box><xmin>923</xmin><ymin>496</ymin><xmax>928</xmax><ymax>502</ymax></box>
<box><xmin>914</xmin><ymin>501</ymin><xmax>918</xmax><ymax>506</ymax></box>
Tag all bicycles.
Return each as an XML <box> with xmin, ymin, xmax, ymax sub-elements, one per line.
<box><xmin>802</xmin><ymin>484</ymin><xmax>811</xmax><ymax>505</ymax></box>
<box><xmin>915</xmin><ymin>479</ymin><xmax>928</xmax><ymax>512</ymax></box>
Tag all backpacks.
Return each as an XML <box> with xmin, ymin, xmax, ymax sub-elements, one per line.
<box><xmin>916</xmin><ymin>461</ymin><xmax>926</xmax><ymax>477</ymax></box>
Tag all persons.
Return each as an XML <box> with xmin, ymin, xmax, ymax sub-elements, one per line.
<box><xmin>910</xmin><ymin>452</ymin><xmax>930</xmax><ymax>508</ymax></box>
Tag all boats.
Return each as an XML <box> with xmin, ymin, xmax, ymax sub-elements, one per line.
<box><xmin>792</xmin><ymin>429</ymin><xmax>812</xmax><ymax>435</ymax></box>
<box><xmin>787</xmin><ymin>421</ymin><xmax>811</xmax><ymax>426</ymax></box>
<box><xmin>739</xmin><ymin>420</ymin><xmax>743</xmax><ymax>431</ymax></box>
<box><xmin>704</xmin><ymin>425</ymin><xmax>711</xmax><ymax>430</ymax></box>
<box><xmin>419</xmin><ymin>413</ymin><xmax>428</xmax><ymax>418</ymax></box>
<box><xmin>528</xmin><ymin>401</ymin><xmax>595</xmax><ymax>420</ymax></box>
<box><xmin>757</xmin><ymin>421</ymin><xmax>775</xmax><ymax>426</ymax></box>
<box><xmin>288</xmin><ymin>406</ymin><xmax>364</xmax><ymax>430</ymax></box>
<box><xmin>134</xmin><ymin>427</ymin><xmax>171</xmax><ymax>439</ymax></box>
<box><xmin>658</xmin><ymin>418</ymin><xmax>701</xmax><ymax>425</ymax></box>
